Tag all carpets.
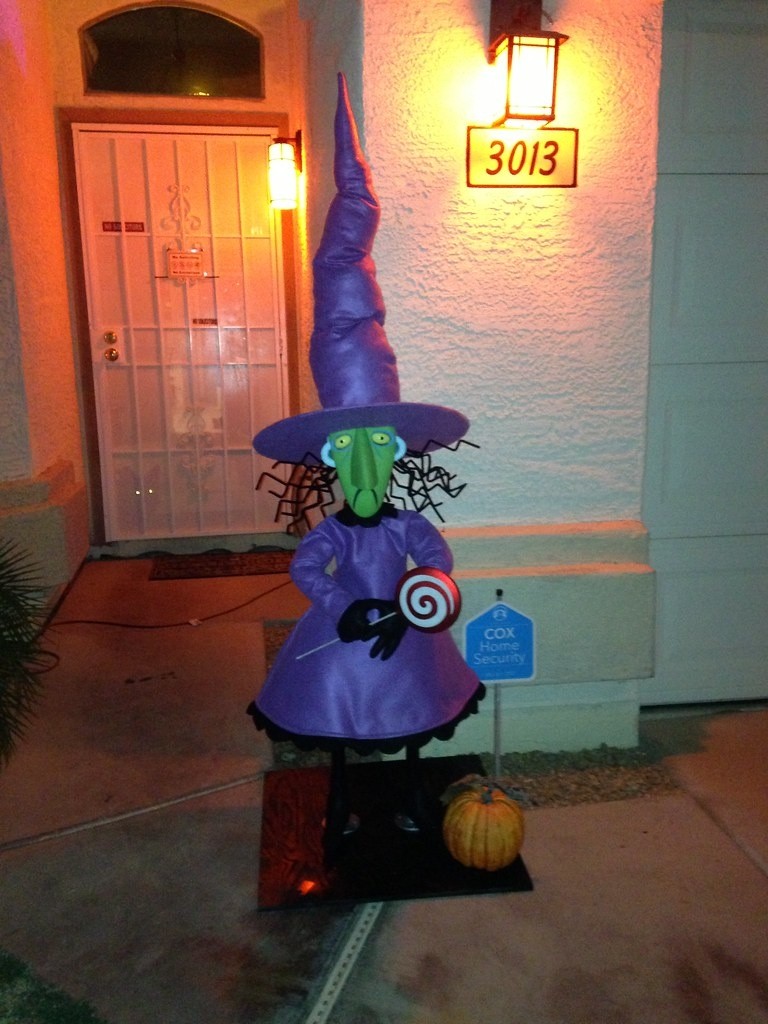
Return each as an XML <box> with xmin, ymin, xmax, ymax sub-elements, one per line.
<box><xmin>261</xmin><ymin>615</ymin><xmax>380</xmax><ymax>767</ymax></box>
<box><xmin>148</xmin><ymin>548</ymin><xmax>294</xmax><ymax>580</ymax></box>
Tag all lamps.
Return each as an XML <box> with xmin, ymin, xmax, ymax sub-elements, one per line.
<box><xmin>487</xmin><ymin>1</ymin><xmax>570</xmax><ymax>130</ymax></box>
<box><xmin>267</xmin><ymin>129</ymin><xmax>304</xmax><ymax>211</ymax></box>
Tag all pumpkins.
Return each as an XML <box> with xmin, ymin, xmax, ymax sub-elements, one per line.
<box><xmin>442</xmin><ymin>785</ymin><xmax>524</xmax><ymax>871</ymax></box>
